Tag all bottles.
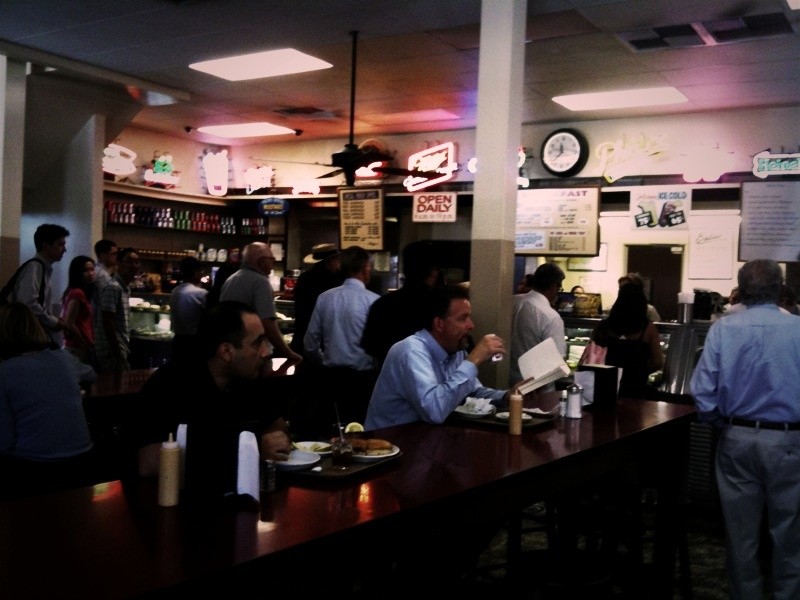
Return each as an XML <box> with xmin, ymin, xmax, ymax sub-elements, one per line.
<box><xmin>567</xmin><ymin>383</ymin><xmax>584</xmax><ymax>418</ymax></box>
<box><xmin>166</xmin><ymin>263</ymin><xmax>173</xmax><ymax>281</ymax></box>
<box><xmin>242</xmin><ymin>218</ymin><xmax>265</xmax><ymax>235</ymax></box>
<box><xmin>509</xmin><ymin>390</ymin><xmax>523</xmax><ymax>435</ymax></box>
<box><xmin>159</xmin><ymin>432</ymin><xmax>182</xmax><ymax>506</ymax></box>
<box><xmin>135</xmin><ymin>273</ymin><xmax>143</xmax><ymax>288</ymax></box>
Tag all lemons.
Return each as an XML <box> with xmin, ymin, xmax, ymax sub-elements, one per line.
<box><xmin>344</xmin><ymin>422</ymin><xmax>364</xmax><ymax>432</ymax></box>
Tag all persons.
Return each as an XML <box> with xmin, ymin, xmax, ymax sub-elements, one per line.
<box><xmin>0</xmin><ymin>223</ymin><xmax>800</xmax><ymax>600</ymax></box>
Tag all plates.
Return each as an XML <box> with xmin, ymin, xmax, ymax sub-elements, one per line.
<box><xmin>294</xmin><ymin>442</ymin><xmax>337</xmax><ymax>454</ymax></box>
<box><xmin>270</xmin><ymin>452</ymin><xmax>321</xmax><ymax>469</ymax></box>
<box><xmin>455</xmin><ymin>406</ymin><xmax>492</xmax><ymax>418</ymax></box>
<box><xmin>353</xmin><ymin>446</ymin><xmax>400</xmax><ymax>462</ymax></box>
<box><xmin>495</xmin><ymin>412</ymin><xmax>532</xmax><ymax>420</ymax></box>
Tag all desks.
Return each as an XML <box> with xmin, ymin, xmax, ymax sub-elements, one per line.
<box><xmin>0</xmin><ymin>357</ymin><xmax>704</xmax><ymax>600</ymax></box>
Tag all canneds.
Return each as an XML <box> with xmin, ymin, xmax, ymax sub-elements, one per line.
<box><xmin>195</xmin><ymin>241</ymin><xmax>207</xmax><ymax>260</ymax></box>
<box><xmin>106</xmin><ymin>200</ymin><xmax>265</xmax><ymax>234</ymax></box>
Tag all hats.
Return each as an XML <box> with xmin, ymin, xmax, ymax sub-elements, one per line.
<box><xmin>302</xmin><ymin>244</ymin><xmax>342</xmax><ymax>264</ymax></box>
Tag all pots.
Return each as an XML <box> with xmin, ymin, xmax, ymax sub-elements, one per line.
<box><xmin>128</xmin><ymin>306</ymin><xmax>172</xmax><ymax>332</ymax></box>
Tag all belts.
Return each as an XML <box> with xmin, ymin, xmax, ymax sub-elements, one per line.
<box><xmin>724</xmin><ymin>417</ymin><xmax>800</xmax><ymax>430</ymax></box>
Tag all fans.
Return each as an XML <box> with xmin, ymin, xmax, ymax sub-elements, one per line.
<box><xmin>247</xmin><ymin>31</ymin><xmax>446</xmax><ymax>184</ymax></box>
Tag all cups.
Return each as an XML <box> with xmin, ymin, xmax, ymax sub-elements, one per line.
<box><xmin>374</xmin><ymin>252</ymin><xmax>390</xmax><ymax>271</ymax></box>
<box><xmin>678</xmin><ymin>303</ymin><xmax>692</xmax><ymax>323</ymax></box>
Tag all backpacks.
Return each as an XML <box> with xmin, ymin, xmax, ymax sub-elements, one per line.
<box><xmin>0</xmin><ymin>258</ymin><xmax>45</xmax><ymax>336</ymax></box>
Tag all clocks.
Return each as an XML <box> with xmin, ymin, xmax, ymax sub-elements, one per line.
<box><xmin>538</xmin><ymin>127</ymin><xmax>591</xmax><ymax>177</ymax></box>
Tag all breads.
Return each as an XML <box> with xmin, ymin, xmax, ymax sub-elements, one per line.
<box><xmin>349</xmin><ymin>438</ymin><xmax>392</xmax><ymax>452</ymax></box>
